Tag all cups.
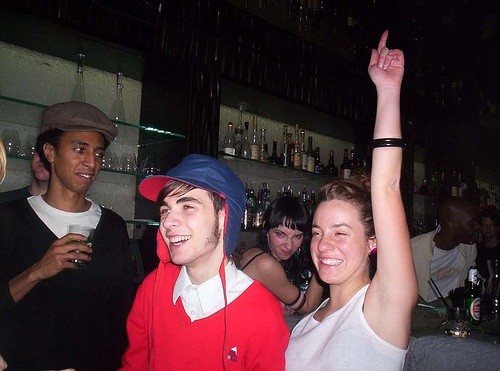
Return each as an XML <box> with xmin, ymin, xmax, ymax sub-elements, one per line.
<box><xmin>67</xmin><ymin>225</ymin><xmax>95</xmax><ymax>266</ymax></box>
<box><xmin>445</xmin><ymin>306</ymin><xmax>471</xmax><ymax>338</ymax></box>
<box><xmin>0</xmin><ymin>129</ymin><xmax>162</xmax><ymax>176</ymax></box>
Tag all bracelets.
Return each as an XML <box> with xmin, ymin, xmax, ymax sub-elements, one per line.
<box><xmin>369</xmin><ymin>138</ymin><xmax>405</xmax><ymax>149</ymax></box>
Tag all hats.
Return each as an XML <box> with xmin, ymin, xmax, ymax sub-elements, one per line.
<box><xmin>137</xmin><ymin>154</ymin><xmax>247</xmax><ymax>264</ymax></box>
<box><xmin>40</xmin><ymin>101</ymin><xmax>119</xmax><ymax>143</ymax></box>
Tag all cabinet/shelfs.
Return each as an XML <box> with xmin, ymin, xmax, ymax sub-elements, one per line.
<box><xmin>0</xmin><ymin>0</ymin><xmax>500</xmax><ymax>248</ymax></box>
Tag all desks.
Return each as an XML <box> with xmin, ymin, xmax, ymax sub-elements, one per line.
<box><xmin>284</xmin><ymin>296</ymin><xmax>500</xmax><ymax>371</ymax></box>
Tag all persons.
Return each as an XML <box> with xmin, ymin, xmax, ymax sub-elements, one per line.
<box><xmin>1</xmin><ymin>148</ymin><xmax>49</xmax><ymax>202</ymax></box>
<box><xmin>235</xmin><ymin>195</ymin><xmax>326</xmax><ymax>316</ymax></box>
<box><xmin>477</xmin><ymin>205</ymin><xmax>500</xmax><ymax>305</ymax></box>
<box><xmin>1</xmin><ymin>101</ymin><xmax>136</xmax><ymax>371</ymax></box>
<box><xmin>284</xmin><ymin>28</ymin><xmax>419</xmax><ymax>370</ymax></box>
<box><xmin>118</xmin><ymin>153</ymin><xmax>290</xmax><ymax>371</ymax></box>
<box><xmin>410</xmin><ymin>196</ymin><xmax>482</xmax><ymax>302</ymax></box>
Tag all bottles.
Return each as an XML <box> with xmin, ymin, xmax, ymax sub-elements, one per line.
<box><xmin>112</xmin><ymin>72</ymin><xmax>125</xmax><ymax>121</ymax></box>
<box><xmin>272</xmin><ymin>125</ymin><xmax>306</xmax><ymax>171</ymax></box>
<box><xmin>350</xmin><ymin>149</ymin><xmax>355</xmax><ymax>165</ymax></box>
<box><xmin>325</xmin><ymin>150</ymin><xmax>337</xmax><ymax>176</ymax></box>
<box><xmin>234</xmin><ymin>113</ymin><xmax>244</xmax><ymax>157</ymax></box>
<box><xmin>464</xmin><ymin>265</ymin><xmax>480</xmax><ymax>326</ymax></box>
<box><xmin>71</xmin><ymin>54</ymin><xmax>86</xmax><ymax>102</ymax></box>
<box><xmin>258</xmin><ymin>129</ymin><xmax>270</xmax><ymax>162</ymax></box>
<box><xmin>307</xmin><ymin>137</ymin><xmax>315</xmax><ymax>172</ymax></box>
<box><xmin>340</xmin><ymin>149</ymin><xmax>351</xmax><ymax>179</ymax></box>
<box><xmin>240</xmin><ymin>122</ymin><xmax>252</xmax><ymax>159</ymax></box>
<box><xmin>240</xmin><ymin>182</ymin><xmax>316</xmax><ymax>232</ymax></box>
<box><xmin>224</xmin><ymin>122</ymin><xmax>234</xmax><ymax>148</ymax></box>
<box><xmin>421</xmin><ymin>169</ymin><xmax>500</xmax><ymax>210</ymax></box>
<box><xmin>315</xmin><ymin>147</ymin><xmax>324</xmax><ymax>173</ymax></box>
<box><xmin>250</xmin><ymin>130</ymin><xmax>259</xmax><ymax>160</ymax></box>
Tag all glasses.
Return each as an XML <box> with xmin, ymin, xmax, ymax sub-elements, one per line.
<box><xmin>482</xmin><ymin>224</ymin><xmax>497</xmax><ymax>228</ymax></box>
